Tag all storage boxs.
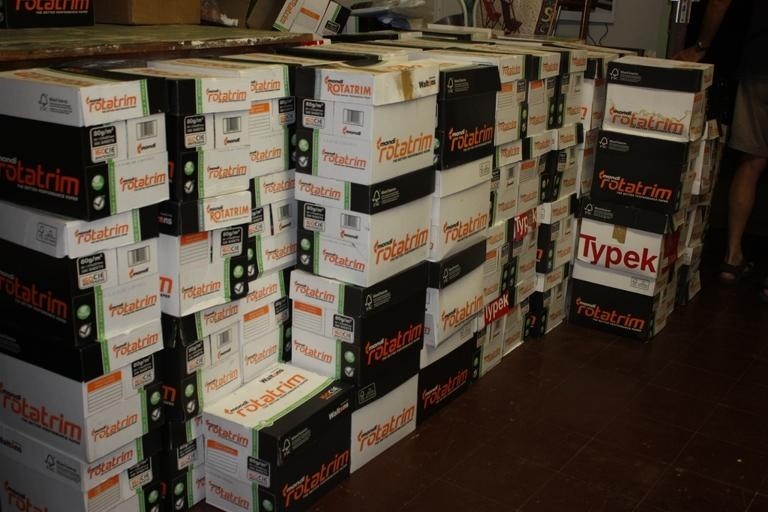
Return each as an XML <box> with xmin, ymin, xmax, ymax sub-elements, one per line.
<box><xmin>2</xmin><ymin>27</ymin><xmax>564</xmax><ymax>512</ymax></box>
<box><xmin>541</xmin><ymin>33</ymin><xmax>723</xmax><ymax>339</ymax></box>
<box><xmin>274</xmin><ymin>1</ymin><xmax>349</xmax><ymax>38</ymax></box>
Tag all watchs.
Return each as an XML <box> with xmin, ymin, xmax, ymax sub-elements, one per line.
<box><xmin>693</xmin><ymin>39</ymin><xmax>712</xmax><ymax>54</ymax></box>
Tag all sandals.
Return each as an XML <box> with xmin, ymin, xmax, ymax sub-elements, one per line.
<box><xmin>719</xmin><ymin>256</ymin><xmax>755</xmax><ymax>288</ymax></box>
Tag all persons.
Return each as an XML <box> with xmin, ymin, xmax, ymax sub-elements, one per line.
<box><xmin>667</xmin><ymin>1</ymin><xmax>768</xmax><ymax>286</ymax></box>
